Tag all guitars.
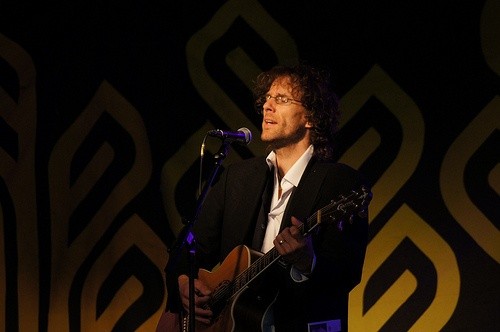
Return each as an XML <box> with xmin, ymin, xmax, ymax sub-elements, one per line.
<box><xmin>155</xmin><ymin>186</ymin><xmax>374</xmax><ymax>332</ymax></box>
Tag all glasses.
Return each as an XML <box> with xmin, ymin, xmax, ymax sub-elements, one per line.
<box><xmin>265</xmin><ymin>94</ymin><xmax>309</xmax><ymax>107</ymax></box>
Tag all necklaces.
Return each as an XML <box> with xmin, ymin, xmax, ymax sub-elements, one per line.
<box><xmin>278</xmin><ymin>172</ymin><xmax>283</xmax><ymax>191</ymax></box>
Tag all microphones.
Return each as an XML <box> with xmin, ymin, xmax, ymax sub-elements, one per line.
<box><xmin>208</xmin><ymin>127</ymin><xmax>252</xmax><ymax>145</ymax></box>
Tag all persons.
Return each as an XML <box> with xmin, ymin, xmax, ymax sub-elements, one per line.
<box><xmin>164</xmin><ymin>61</ymin><xmax>369</xmax><ymax>332</ymax></box>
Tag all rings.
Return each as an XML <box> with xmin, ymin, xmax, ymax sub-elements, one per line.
<box><xmin>278</xmin><ymin>240</ymin><xmax>285</xmax><ymax>244</ymax></box>
